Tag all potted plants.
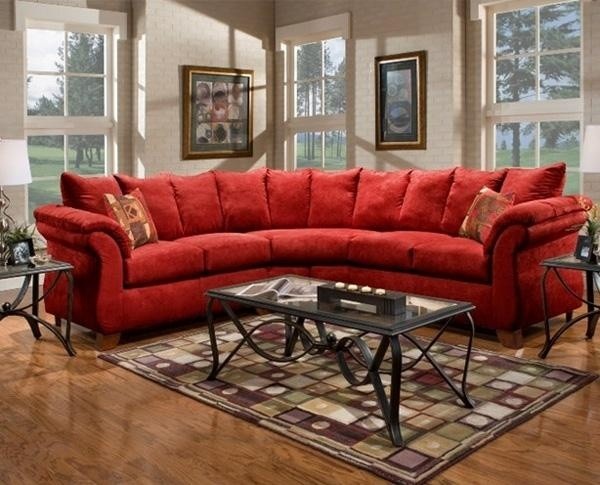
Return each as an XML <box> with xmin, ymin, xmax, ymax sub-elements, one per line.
<box><xmin>573</xmin><ymin>217</ymin><xmax>600</xmax><ymax>265</ymax></box>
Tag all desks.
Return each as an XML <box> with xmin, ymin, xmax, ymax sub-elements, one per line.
<box><xmin>538</xmin><ymin>252</ymin><xmax>600</xmax><ymax>359</ymax></box>
<box><xmin>0</xmin><ymin>255</ymin><xmax>77</xmax><ymax>357</ymax></box>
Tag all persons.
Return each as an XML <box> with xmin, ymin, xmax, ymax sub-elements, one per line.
<box><xmin>196</xmin><ymin>80</ymin><xmax>243</xmax><ymax>120</ymax></box>
<box><xmin>16</xmin><ymin>248</ymin><xmax>24</xmax><ymax>261</ymax></box>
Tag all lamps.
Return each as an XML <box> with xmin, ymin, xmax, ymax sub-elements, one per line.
<box><xmin>0</xmin><ymin>138</ymin><xmax>32</xmax><ymax>265</ymax></box>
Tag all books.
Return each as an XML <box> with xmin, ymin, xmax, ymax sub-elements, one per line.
<box><xmin>234</xmin><ymin>277</ymin><xmax>327</xmax><ymax>302</ymax></box>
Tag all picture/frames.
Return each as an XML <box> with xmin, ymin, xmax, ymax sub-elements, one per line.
<box><xmin>375</xmin><ymin>49</ymin><xmax>426</xmax><ymax>150</ymax></box>
<box><xmin>573</xmin><ymin>236</ymin><xmax>594</xmax><ymax>262</ymax></box>
<box><xmin>10</xmin><ymin>238</ymin><xmax>35</xmax><ymax>266</ymax></box>
<box><xmin>182</xmin><ymin>65</ymin><xmax>254</xmax><ymax>159</ymax></box>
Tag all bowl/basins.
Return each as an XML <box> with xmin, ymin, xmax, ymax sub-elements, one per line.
<box><xmin>30</xmin><ymin>255</ymin><xmax>52</xmax><ymax>267</ymax></box>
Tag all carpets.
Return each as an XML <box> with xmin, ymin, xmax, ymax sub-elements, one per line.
<box><xmin>97</xmin><ymin>313</ymin><xmax>600</xmax><ymax>485</ymax></box>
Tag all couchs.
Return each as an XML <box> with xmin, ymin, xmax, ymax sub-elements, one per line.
<box><xmin>34</xmin><ymin>195</ymin><xmax>593</xmax><ymax>349</ymax></box>
